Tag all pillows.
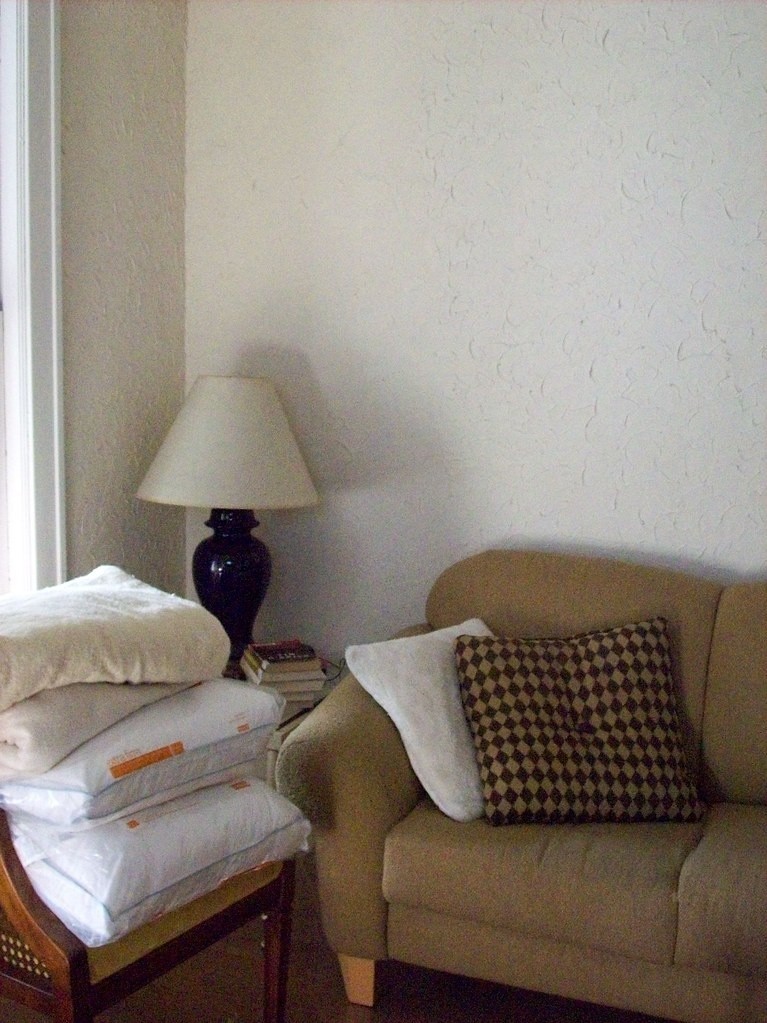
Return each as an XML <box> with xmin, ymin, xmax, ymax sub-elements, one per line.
<box><xmin>344</xmin><ymin>620</ymin><xmax>501</xmax><ymax>825</ymax></box>
<box><xmin>454</xmin><ymin>618</ymin><xmax>702</xmax><ymax>817</ymax></box>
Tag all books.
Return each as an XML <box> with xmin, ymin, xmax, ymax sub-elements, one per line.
<box><xmin>239</xmin><ymin>638</ymin><xmax>326</xmax><ymax>727</ymax></box>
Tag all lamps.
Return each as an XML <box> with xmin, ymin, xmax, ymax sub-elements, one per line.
<box><xmin>137</xmin><ymin>375</ymin><xmax>319</xmax><ymax>680</ymax></box>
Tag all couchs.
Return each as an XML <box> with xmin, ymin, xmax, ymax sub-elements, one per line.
<box><xmin>276</xmin><ymin>545</ymin><xmax>767</xmax><ymax>1023</ymax></box>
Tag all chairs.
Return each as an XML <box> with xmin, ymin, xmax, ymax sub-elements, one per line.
<box><xmin>0</xmin><ymin>815</ymin><xmax>290</xmax><ymax>1023</ymax></box>
